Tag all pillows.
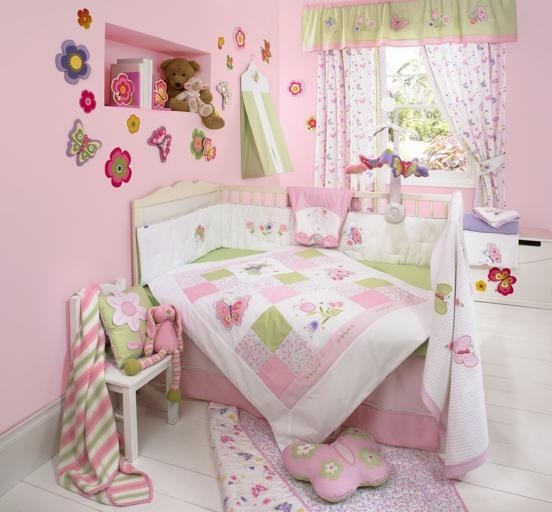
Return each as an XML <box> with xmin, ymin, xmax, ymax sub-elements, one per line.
<box><xmin>282</xmin><ymin>426</ymin><xmax>392</xmax><ymax>503</ymax></box>
<box><xmin>97</xmin><ymin>284</ymin><xmax>160</xmax><ymax>369</ymax></box>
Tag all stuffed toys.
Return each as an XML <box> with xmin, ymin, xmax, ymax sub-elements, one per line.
<box><xmin>121</xmin><ymin>303</ymin><xmax>186</xmax><ymax>404</ymax></box>
<box><xmin>175</xmin><ymin>77</ymin><xmax>214</xmax><ymax>119</ymax></box>
<box><xmin>153</xmin><ymin>79</ymin><xmax>169</xmax><ymax>110</ymax></box>
<box><xmin>159</xmin><ymin>57</ymin><xmax>225</xmax><ymax>130</ymax></box>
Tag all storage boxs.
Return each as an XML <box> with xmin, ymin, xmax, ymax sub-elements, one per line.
<box><xmin>464</xmin><ymin>230</ymin><xmax>552</xmax><ymax>309</ymax></box>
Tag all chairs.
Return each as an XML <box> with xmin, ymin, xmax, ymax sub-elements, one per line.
<box><xmin>68</xmin><ymin>278</ymin><xmax>185</xmax><ymax>461</ymax></box>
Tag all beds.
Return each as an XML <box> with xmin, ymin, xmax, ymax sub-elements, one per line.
<box><xmin>129</xmin><ymin>179</ymin><xmax>462</xmax><ymax>450</ymax></box>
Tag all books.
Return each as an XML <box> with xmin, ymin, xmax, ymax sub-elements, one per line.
<box><xmin>108</xmin><ymin>56</ymin><xmax>153</xmax><ymax>110</ymax></box>
